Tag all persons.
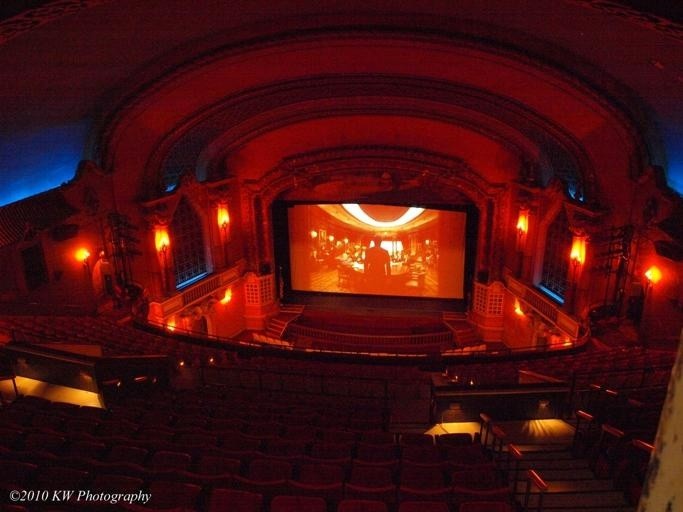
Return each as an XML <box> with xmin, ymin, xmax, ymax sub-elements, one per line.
<box><xmin>363</xmin><ymin>232</ymin><xmax>391</xmax><ymax>295</ymax></box>
<box><xmin>307</xmin><ymin>241</ymin><xmax>438</xmax><ymax>280</ymax></box>
